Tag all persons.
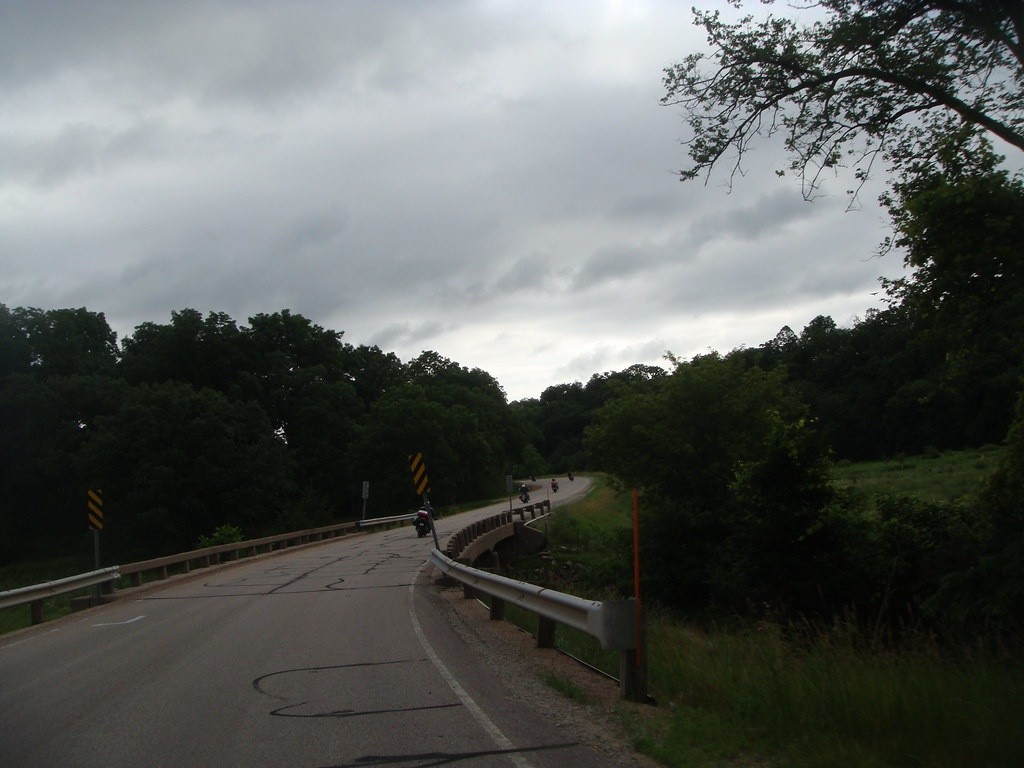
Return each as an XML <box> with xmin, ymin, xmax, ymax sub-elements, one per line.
<box><xmin>520</xmin><ymin>484</ymin><xmax>528</xmax><ymax>493</ymax></box>
<box><xmin>421</xmin><ymin>501</ymin><xmax>437</xmax><ymax>532</ymax></box>
<box><xmin>568</xmin><ymin>471</ymin><xmax>572</xmax><ymax>479</ymax></box>
<box><xmin>551</xmin><ymin>479</ymin><xmax>559</xmax><ymax>489</ymax></box>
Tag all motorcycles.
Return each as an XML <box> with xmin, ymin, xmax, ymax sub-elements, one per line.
<box><xmin>415</xmin><ymin>510</ymin><xmax>434</xmax><ymax>538</ymax></box>
<box><xmin>518</xmin><ymin>488</ymin><xmax>531</xmax><ymax>503</ymax></box>
<box><xmin>552</xmin><ymin>483</ymin><xmax>559</xmax><ymax>492</ymax></box>
<box><xmin>568</xmin><ymin>474</ymin><xmax>574</xmax><ymax>481</ymax></box>
